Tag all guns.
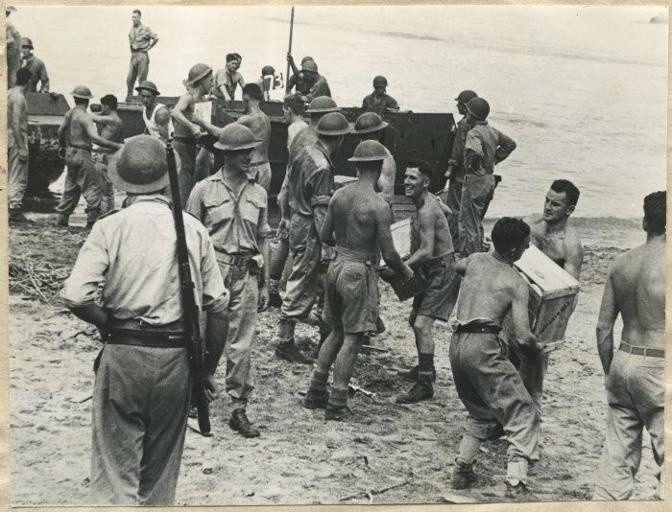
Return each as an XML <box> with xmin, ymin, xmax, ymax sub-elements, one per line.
<box><xmin>165</xmin><ymin>143</ymin><xmax>210</xmax><ymax>433</ymax></box>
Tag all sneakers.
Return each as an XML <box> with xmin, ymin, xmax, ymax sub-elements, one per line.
<box><xmin>8</xmin><ymin>207</ymin><xmax>36</xmax><ymax>227</ymax></box>
<box><xmin>504</xmin><ymin>480</ymin><xmax>542</xmax><ymax>502</ymax></box>
<box><xmin>85</xmin><ymin>207</ymin><xmax>101</xmax><ymax>229</ymax></box>
<box><xmin>188</xmin><ymin>401</ymin><xmax>200</xmax><ymax>418</ymax></box>
<box><xmin>57</xmin><ymin>213</ymin><xmax>69</xmax><ymax>228</ymax></box>
<box><xmin>266</xmin><ymin>273</ymin><xmax>325</xmax><ymax>325</ymax></box>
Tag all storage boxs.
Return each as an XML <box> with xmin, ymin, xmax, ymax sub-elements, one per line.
<box><xmin>195</xmin><ymin>102</ymin><xmax>236</xmax><ymax>153</ymax></box>
<box><xmin>380</xmin><ymin>218</ymin><xmax>425</xmax><ymax>302</ymax></box>
<box><xmin>514</xmin><ymin>243</ymin><xmax>580</xmax><ymax>344</ymax></box>
<box><xmin>333</xmin><ymin>175</ymin><xmax>357</xmax><ymax>189</ymax></box>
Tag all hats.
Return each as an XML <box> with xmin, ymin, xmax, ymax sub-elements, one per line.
<box><xmin>349</xmin><ymin>111</ymin><xmax>390</xmax><ymax>135</ymax></box>
<box><xmin>304</xmin><ymin>96</ymin><xmax>343</xmax><ymax>114</ymax></box>
<box><xmin>346</xmin><ymin>140</ymin><xmax>395</xmax><ymax>163</ymax></box>
<box><xmin>135</xmin><ymin>81</ymin><xmax>161</xmax><ymax>95</ymax></box>
<box><xmin>314</xmin><ymin>112</ymin><xmax>355</xmax><ymax>136</ymax></box>
<box><xmin>213</xmin><ymin>122</ymin><xmax>265</xmax><ymax>152</ymax></box>
<box><xmin>22</xmin><ymin>37</ymin><xmax>34</xmax><ymax>51</ymax></box>
<box><xmin>70</xmin><ymin>85</ymin><xmax>94</xmax><ymax>100</ymax></box>
<box><xmin>186</xmin><ymin>63</ymin><xmax>214</xmax><ymax>85</ymax></box>
<box><xmin>373</xmin><ymin>75</ymin><xmax>387</xmax><ymax>89</ymax></box>
<box><xmin>454</xmin><ymin>90</ymin><xmax>478</xmax><ymax>106</ymax></box>
<box><xmin>107</xmin><ymin>135</ymin><xmax>182</xmax><ymax>194</ymax></box>
<box><xmin>463</xmin><ymin>97</ymin><xmax>490</xmax><ymax>121</ymax></box>
<box><xmin>300</xmin><ymin>56</ymin><xmax>318</xmax><ymax>74</ymax></box>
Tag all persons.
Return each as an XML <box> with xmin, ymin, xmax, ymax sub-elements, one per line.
<box><xmin>266</xmin><ymin>52</ymin><xmax>517</xmax><ymax>425</ymax></box>
<box><xmin>451</xmin><ymin>179</ymin><xmax>582</xmax><ymax>498</ymax></box>
<box><xmin>592</xmin><ymin>190</ymin><xmax>665</xmax><ymax>499</ymax></box>
<box><xmin>5</xmin><ymin>4</ymin><xmax>57</xmax><ymax>222</ymax></box>
<box><xmin>56</xmin><ymin>134</ymin><xmax>231</xmax><ymax>502</ymax></box>
<box><xmin>54</xmin><ymin>7</ymin><xmax>284</xmax><ymax>438</ymax></box>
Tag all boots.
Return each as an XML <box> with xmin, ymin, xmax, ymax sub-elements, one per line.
<box><xmin>269</xmin><ymin>314</ymin><xmax>314</xmax><ymax>365</ymax></box>
<box><xmin>325</xmin><ymin>383</ymin><xmax>374</xmax><ymax>426</ymax></box>
<box><xmin>394</xmin><ymin>352</ymin><xmax>437</xmax><ymax>404</ymax></box>
<box><xmin>451</xmin><ymin>456</ymin><xmax>497</xmax><ymax>490</ymax></box>
<box><xmin>228</xmin><ymin>396</ymin><xmax>261</xmax><ymax>438</ymax></box>
<box><xmin>312</xmin><ymin>314</ymin><xmax>333</xmax><ymax>360</ymax></box>
<box><xmin>302</xmin><ymin>369</ymin><xmax>330</xmax><ymax>410</ymax></box>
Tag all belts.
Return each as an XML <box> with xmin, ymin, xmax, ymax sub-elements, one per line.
<box><xmin>455</xmin><ymin>325</ymin><xmax>502</xmax><ymax>337</ymax></box>
<box><xmin>103</xmin><ymin>329</ymin><xmax>194</xmax><ymax>350</ymax></box>
<box><xmin>618</xmin><ymin>342</ymin><xmax>665</xmax><ymax>359</ymax></box>
<box><xmin>252</xmin><ymin>158</ymin><xmax>270</xmax><ymax>168</ymax></box>
